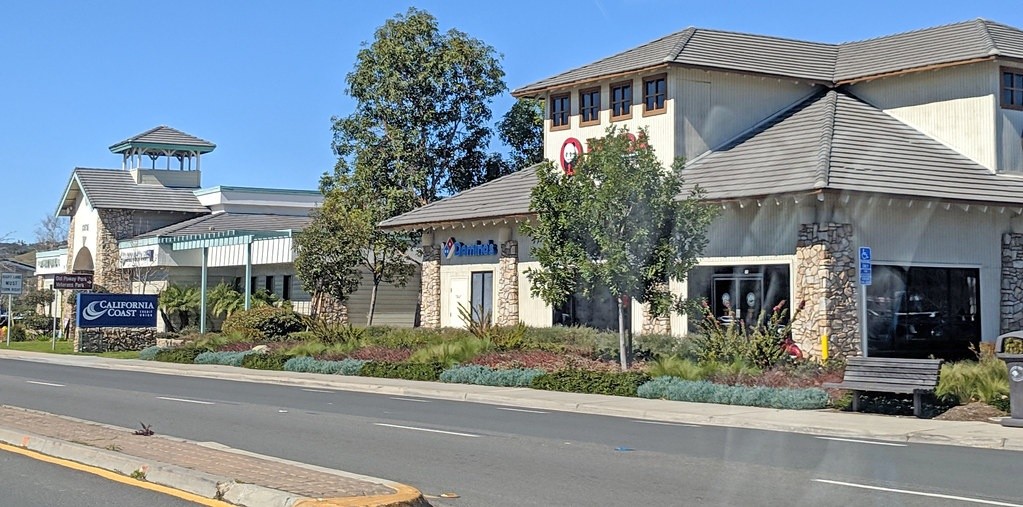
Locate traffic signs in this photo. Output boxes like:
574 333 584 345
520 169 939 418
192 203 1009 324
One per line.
53 273 93 290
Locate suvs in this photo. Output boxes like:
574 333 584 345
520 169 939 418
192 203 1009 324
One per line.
865 294 950 344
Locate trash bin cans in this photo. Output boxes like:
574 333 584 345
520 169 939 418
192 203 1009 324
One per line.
994 330 1023 428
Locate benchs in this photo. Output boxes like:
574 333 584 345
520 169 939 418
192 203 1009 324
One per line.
822 357 944 415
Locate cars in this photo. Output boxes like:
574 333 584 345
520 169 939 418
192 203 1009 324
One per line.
929 317 982 349
